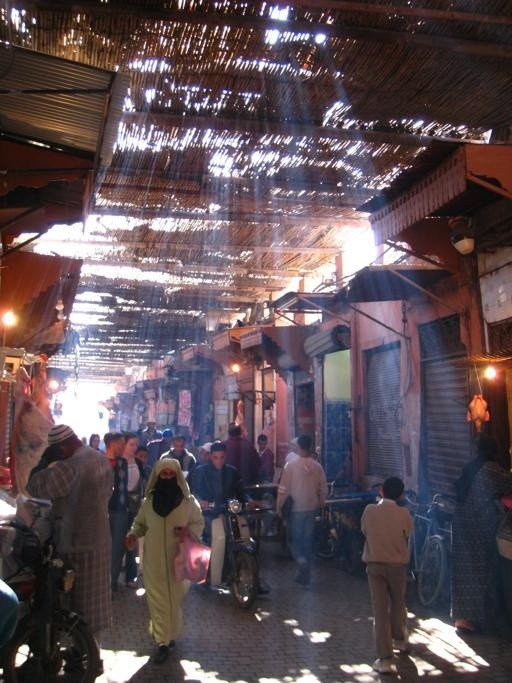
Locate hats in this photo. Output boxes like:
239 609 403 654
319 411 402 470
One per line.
197 442 213 454
48 425 75 445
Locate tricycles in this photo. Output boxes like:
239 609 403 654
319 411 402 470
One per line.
250 457 343 560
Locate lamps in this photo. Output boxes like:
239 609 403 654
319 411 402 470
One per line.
483 358 498 381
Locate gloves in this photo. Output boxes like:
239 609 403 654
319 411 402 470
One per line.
41 444 62 463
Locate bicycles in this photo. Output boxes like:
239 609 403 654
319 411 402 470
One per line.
390 487 447 606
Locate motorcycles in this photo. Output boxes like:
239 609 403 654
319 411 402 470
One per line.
1 514 100 682
183 495 273 611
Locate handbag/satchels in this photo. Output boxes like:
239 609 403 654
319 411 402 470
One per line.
282 496 293 518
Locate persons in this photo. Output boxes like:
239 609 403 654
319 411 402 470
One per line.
257 434 275 481
26 425 115 679
359 477 415 674
103 432 144 597
89 420 197 480
220 424 261 485
275 435 328 591
186 441 257 545
122 457 205 665
449 437 512 633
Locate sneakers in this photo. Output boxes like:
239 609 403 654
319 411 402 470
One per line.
256 578 271 593
295 563 310 585
454 618 475 632
373 658 391 674
393 628 409 652
155 640 174 664
